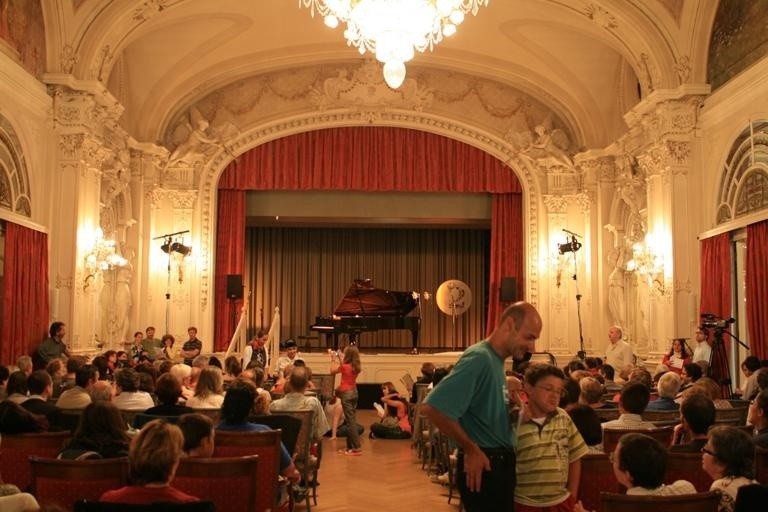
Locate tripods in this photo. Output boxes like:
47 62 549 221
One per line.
707 337 733 400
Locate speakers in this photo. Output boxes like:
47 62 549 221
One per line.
227 275 242 299
501 278 516 301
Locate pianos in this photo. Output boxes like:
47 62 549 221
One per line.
309 279 421 354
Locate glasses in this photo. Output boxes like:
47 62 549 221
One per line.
608 451 618 464
699 443 724 460
531 384 566 398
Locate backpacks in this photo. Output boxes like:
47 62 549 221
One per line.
380 416 402 429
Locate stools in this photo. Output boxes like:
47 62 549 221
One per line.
297 335 319 353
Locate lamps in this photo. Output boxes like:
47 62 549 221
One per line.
556 238 583 256
625 229 666 296
297 0 491 91
83 227 129 291
161 237 190 257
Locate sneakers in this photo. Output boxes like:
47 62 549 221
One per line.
337 444 363 457
436 471 452 484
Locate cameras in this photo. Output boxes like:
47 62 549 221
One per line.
336 352 339 356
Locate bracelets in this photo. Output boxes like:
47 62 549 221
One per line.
330 359 336 363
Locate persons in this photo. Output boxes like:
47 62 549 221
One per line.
411 362 453 403
507 358 765 511
369 391 412 441
324 384 365 441
421 301 542 511
0 321 330 512
376 381 399 423
328 344 364 456
692 325 714 365
605 325 634 377
660 340 691 373
